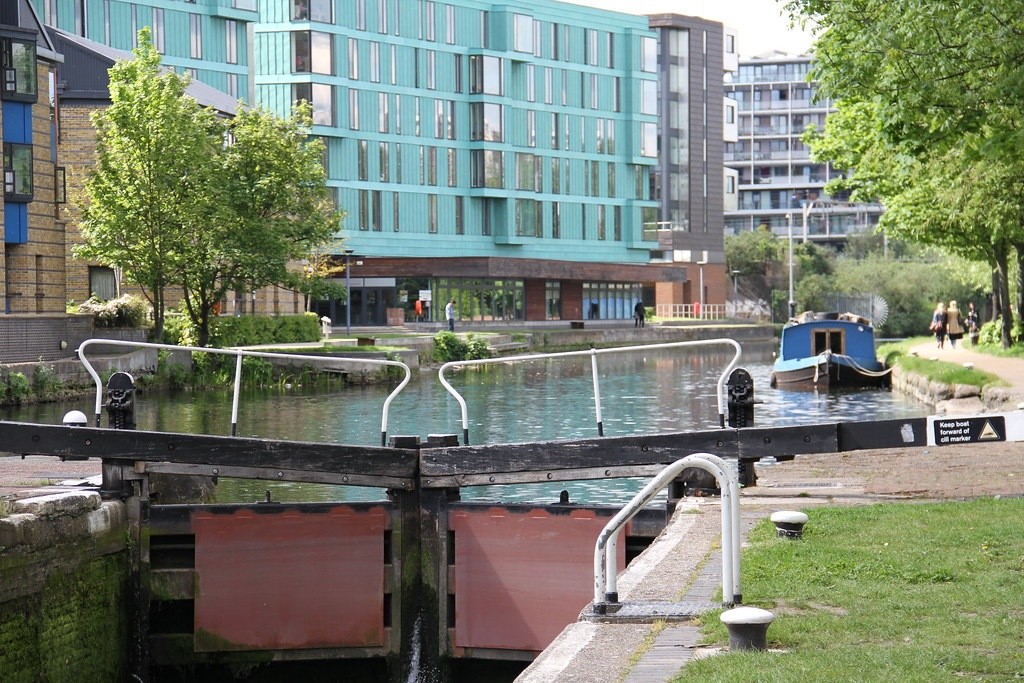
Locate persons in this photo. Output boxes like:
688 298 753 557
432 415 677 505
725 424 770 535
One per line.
635 299 646 328
445 300 456 332
931 299 979 348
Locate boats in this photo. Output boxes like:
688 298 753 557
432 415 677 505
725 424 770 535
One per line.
771 310 892 396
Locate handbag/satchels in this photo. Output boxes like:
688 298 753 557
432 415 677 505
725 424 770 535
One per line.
929 311 942 331
958 308 962 324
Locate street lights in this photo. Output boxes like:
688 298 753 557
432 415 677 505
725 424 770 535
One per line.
732 270 741 318
784 211 797 318
696 261 707 320
343 248 353 336
